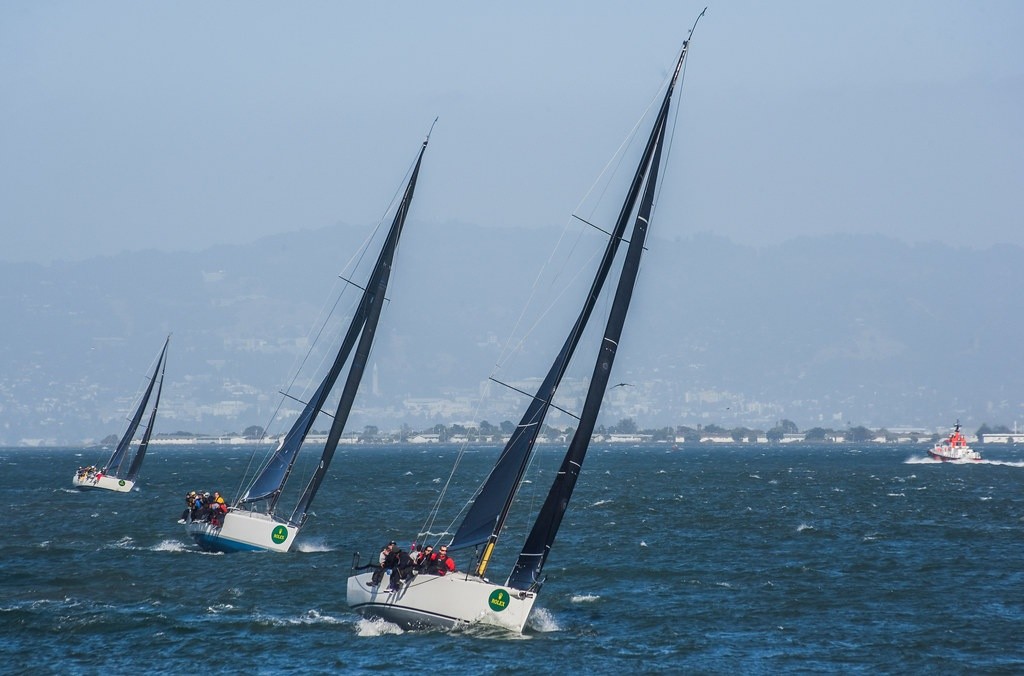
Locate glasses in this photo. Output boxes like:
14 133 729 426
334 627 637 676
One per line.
440 549 447 553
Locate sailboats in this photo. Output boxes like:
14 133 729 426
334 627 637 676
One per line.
348 6 713 638
176 114 440 547
72 333 171 493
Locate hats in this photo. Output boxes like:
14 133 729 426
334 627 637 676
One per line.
388 540 396 545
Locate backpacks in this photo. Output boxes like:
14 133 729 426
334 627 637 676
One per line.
428 554 448 576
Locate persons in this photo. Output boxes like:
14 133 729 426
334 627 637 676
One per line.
75 466 104 486
177 491 227 530
366 541 455 595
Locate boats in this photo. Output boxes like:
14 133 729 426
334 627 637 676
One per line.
926 418 982 461
